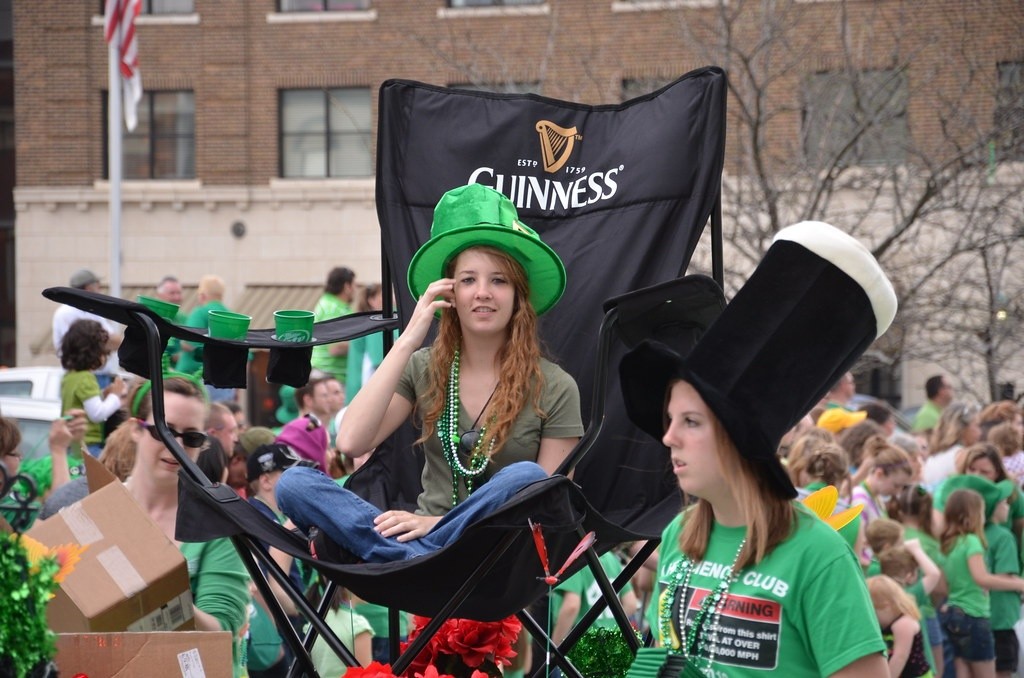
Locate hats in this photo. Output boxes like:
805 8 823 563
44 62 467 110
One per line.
70 269 99 287
619 221 897 502
816 408 867 433
933 475 1014 522
407 184 566 320
239 412 327 483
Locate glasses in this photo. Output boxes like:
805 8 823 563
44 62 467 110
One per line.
137 419 208 448
460 429 486 494
5 451 23 461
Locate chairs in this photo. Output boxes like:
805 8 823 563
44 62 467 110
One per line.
41 66 729 678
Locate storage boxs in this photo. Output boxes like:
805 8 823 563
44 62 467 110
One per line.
53 630 232 678
23 447 196 632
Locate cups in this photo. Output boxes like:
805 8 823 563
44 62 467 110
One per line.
273 310 315 343
137 295 181 324
207 310 252 342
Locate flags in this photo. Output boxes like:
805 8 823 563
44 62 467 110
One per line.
103 0 142 134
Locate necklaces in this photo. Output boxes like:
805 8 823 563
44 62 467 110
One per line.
436 343 496 508
661 537 747 673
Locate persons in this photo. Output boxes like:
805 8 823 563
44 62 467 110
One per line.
645 336 892 678
274 183 585 564
0 268 408 678
776 370 1024 678
503 539 658 678
